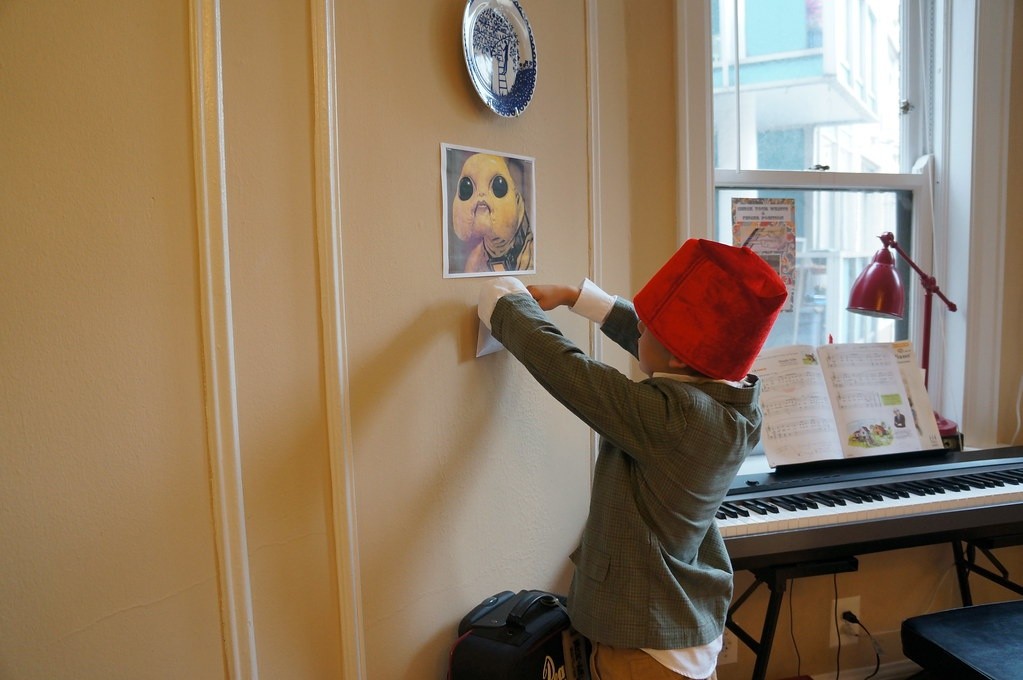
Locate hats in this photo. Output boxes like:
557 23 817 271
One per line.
632 239 788 381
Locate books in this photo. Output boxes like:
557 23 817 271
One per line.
752 339 945 469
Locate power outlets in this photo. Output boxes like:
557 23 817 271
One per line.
717 621 738 666
830 595 861 648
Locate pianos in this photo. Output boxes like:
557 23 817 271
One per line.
712 444 1023 574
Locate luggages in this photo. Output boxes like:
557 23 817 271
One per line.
447 589 592 680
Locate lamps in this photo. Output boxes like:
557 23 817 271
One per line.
845 229 966 452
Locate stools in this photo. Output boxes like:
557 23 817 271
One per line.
900 599 1023 680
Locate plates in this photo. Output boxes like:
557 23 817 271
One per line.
461 0 537 118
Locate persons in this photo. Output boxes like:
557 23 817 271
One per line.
479 240 787 679
